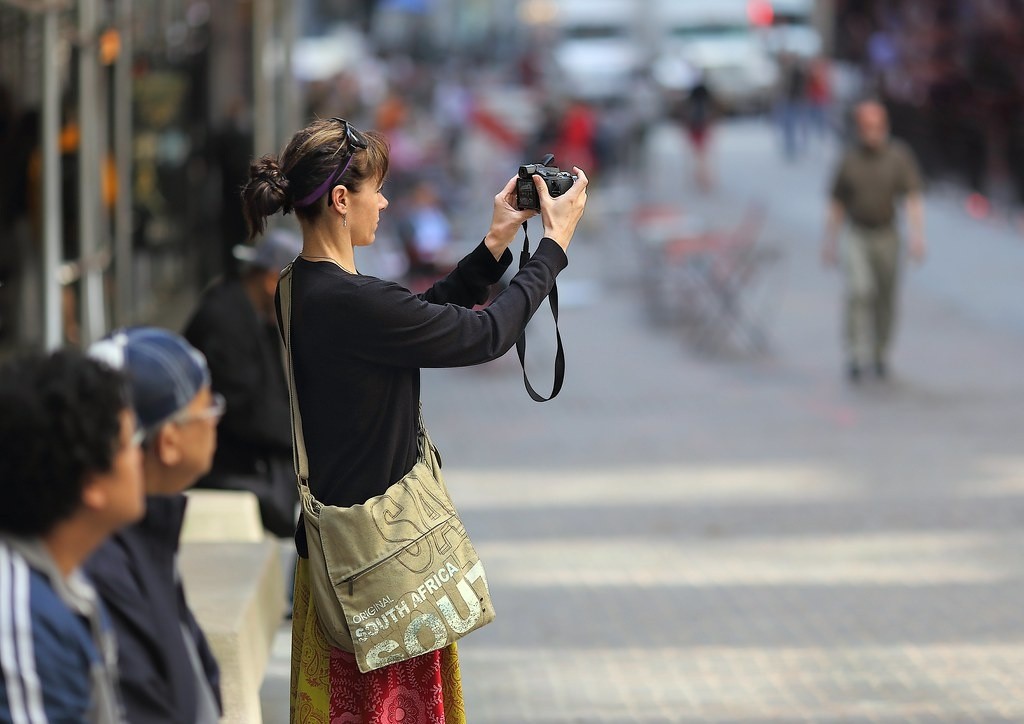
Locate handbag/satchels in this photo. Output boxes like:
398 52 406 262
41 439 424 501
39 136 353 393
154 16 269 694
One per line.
303 461 496 674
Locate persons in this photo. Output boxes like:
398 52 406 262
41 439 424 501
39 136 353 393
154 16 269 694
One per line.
822 100 925 384
182 227 301 538
243 118 588 704
1 350 146 724
78 327 224 723
296 39 807 364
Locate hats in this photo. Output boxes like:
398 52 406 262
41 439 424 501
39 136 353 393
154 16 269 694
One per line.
89 326 207 446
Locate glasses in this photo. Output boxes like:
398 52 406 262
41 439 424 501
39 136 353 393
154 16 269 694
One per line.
177 393 225 422
327 117 368 206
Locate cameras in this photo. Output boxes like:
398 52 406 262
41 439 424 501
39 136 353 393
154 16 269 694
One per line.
516 153 579 213
